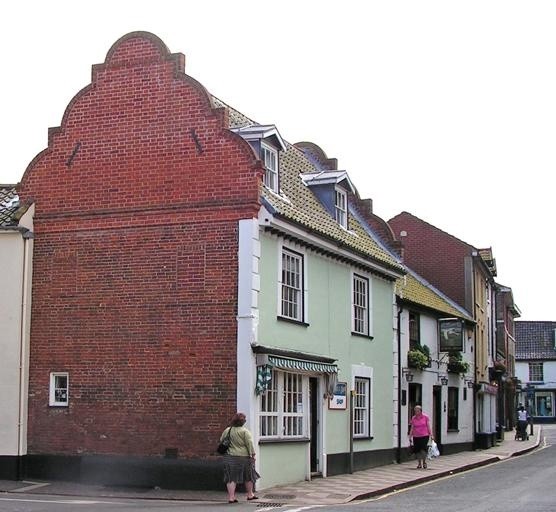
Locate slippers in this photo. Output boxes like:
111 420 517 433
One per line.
229 499 238 503
247 495 258 500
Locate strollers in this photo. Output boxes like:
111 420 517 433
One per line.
514 419 530 441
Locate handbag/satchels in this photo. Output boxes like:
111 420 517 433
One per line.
217 425 232 455
408 440 415 456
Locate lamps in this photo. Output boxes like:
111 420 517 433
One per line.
404 370 414 382
465 378 473 389
438 375 449 386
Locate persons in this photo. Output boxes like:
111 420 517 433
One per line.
219 412 261 503
409 405 432 469
515 401 529 442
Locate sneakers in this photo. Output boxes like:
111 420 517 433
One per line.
417 463 427 468
